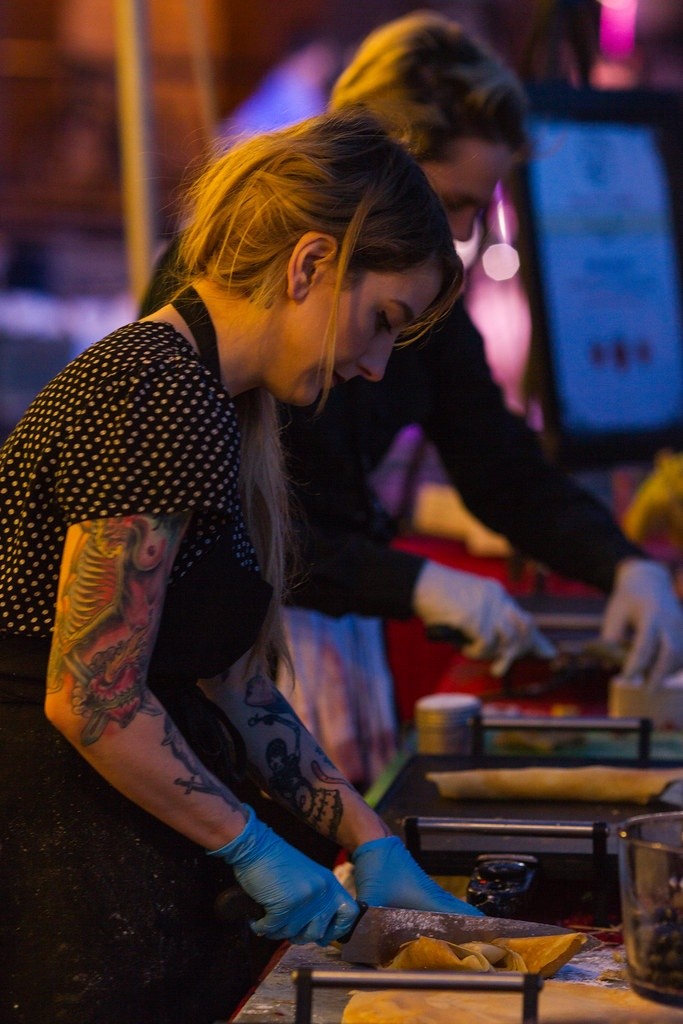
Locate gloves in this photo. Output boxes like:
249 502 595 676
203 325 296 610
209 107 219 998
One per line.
413 559 558 676
602 560 683 693
205 803 359 945
351 836 483 916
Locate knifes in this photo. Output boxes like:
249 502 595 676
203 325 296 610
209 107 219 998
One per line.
338 900 603 966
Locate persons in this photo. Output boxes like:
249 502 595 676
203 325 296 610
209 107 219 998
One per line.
0 116 487 1024
135 9 683 794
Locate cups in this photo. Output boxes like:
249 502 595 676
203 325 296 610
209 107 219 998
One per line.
415 692 485 757
614 809 683 1008
